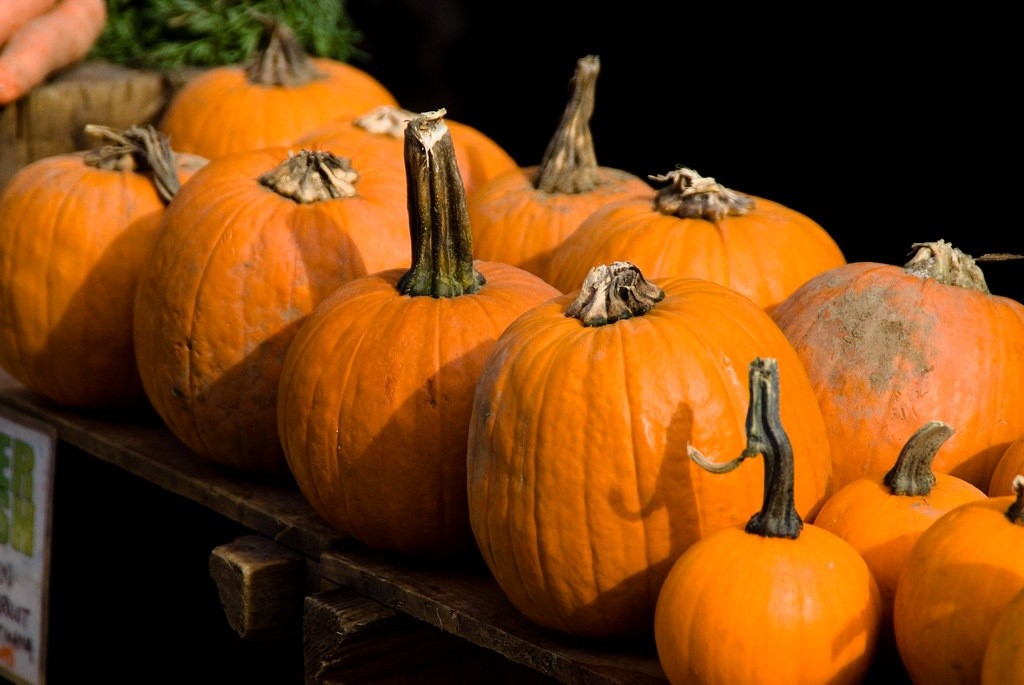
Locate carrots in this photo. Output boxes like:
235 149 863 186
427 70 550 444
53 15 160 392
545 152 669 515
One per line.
0 0 111 105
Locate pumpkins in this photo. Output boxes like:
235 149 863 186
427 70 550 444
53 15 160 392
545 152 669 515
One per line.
1 24 1023 685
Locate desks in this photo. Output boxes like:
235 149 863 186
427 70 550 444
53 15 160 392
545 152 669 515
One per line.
1 383 678 685
